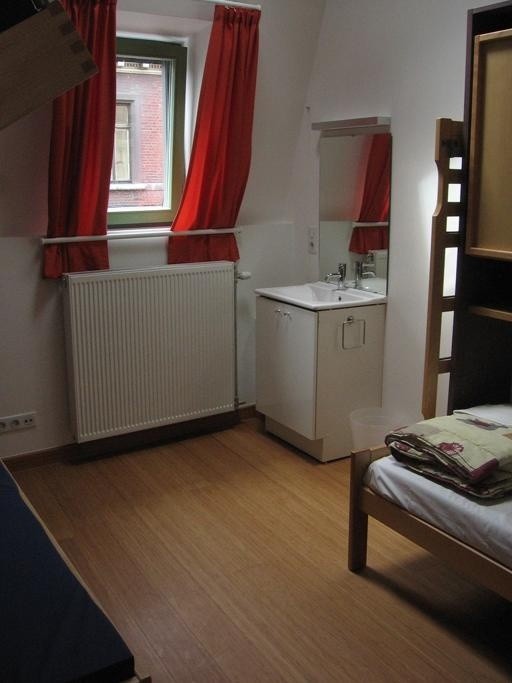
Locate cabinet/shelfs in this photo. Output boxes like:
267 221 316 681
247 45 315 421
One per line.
447 1 512 415
256 296 386 460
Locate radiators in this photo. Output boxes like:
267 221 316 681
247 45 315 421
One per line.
61 261 251 444
366 249 387 278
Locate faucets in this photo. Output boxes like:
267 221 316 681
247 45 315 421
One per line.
325 263 347 289
356 260 377 288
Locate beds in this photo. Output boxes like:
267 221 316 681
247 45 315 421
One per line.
348 445 512 603
1 453 153 683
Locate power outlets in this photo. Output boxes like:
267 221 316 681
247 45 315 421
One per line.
0 411 39 433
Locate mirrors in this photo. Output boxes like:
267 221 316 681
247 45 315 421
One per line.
318 133 391 296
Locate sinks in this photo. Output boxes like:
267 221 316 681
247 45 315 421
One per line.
347 277 386 296
253 283 372 308
